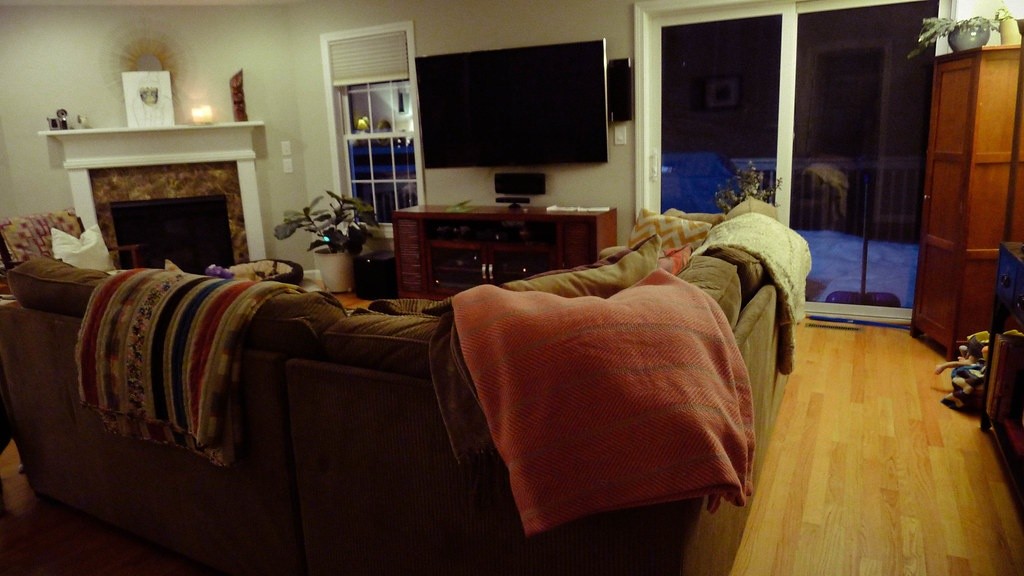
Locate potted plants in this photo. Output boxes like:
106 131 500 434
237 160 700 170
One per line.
271 191 381 293
906 9 1014 60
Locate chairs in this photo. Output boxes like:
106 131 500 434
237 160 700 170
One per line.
0 209 143 295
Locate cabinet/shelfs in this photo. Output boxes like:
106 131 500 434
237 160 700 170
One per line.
392 205 617 300
910 46 1024 362
980 241 1024 530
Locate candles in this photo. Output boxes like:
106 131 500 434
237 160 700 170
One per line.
192 104 212 123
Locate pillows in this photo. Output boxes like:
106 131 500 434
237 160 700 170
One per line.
630 206 712 251
661 208 727 226
49 223 117 273
500 233 661 299
656 244 694 276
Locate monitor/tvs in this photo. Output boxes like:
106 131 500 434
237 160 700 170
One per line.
414 37 608 169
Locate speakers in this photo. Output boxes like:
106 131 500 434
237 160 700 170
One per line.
607 57 632 122
495 173 545 195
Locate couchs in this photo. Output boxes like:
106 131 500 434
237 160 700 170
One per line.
0 199 811 576
